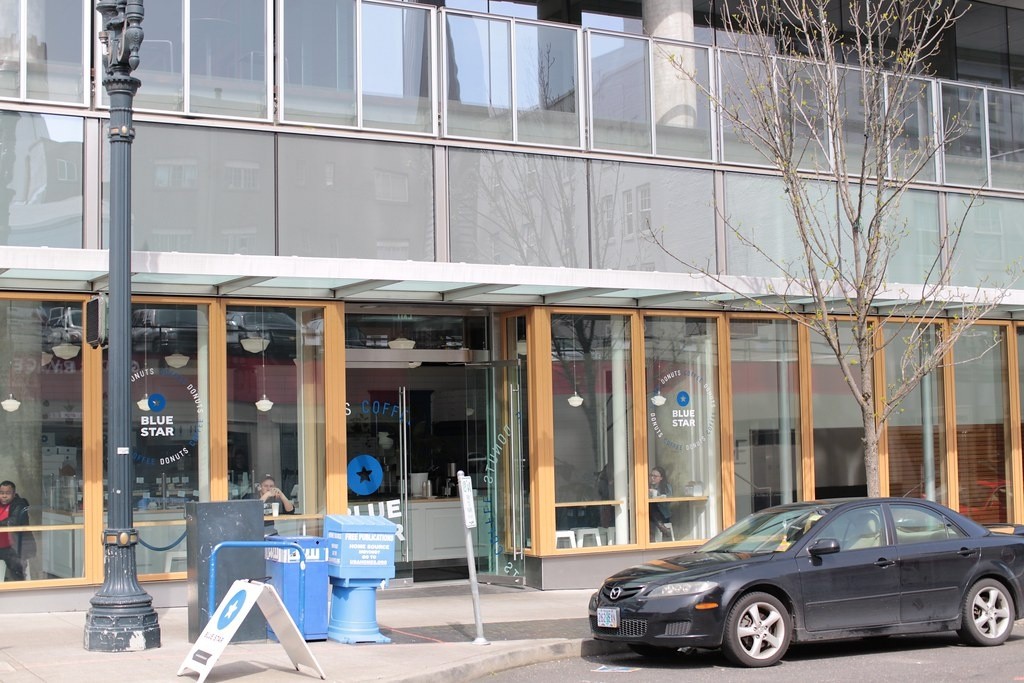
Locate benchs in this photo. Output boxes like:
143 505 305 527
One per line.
895 529 958 544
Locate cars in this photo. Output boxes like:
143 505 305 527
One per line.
587 495 1024 667
45 306 298 365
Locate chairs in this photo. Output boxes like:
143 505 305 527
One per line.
842 514 879 550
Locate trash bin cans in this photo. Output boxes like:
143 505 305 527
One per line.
265 536 328 643
185 500 267 644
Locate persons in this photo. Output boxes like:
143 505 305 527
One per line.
242 477 295 536
596 464 615 528
649 466 673 537
0 480 37 580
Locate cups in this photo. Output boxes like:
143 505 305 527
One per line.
272 503 279 517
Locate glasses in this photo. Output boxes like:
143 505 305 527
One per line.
650 474 661 478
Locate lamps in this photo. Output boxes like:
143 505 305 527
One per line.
164 306 190 368
136 305 155 411
567 318 585 407
651 358 666 406
1 301 21 412
51 304 79 360
240 306 270 353
388 314 415 348
255 307 274 411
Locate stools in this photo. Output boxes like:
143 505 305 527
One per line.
556 523 675 549
164 550 188 574
0 559 32 581
